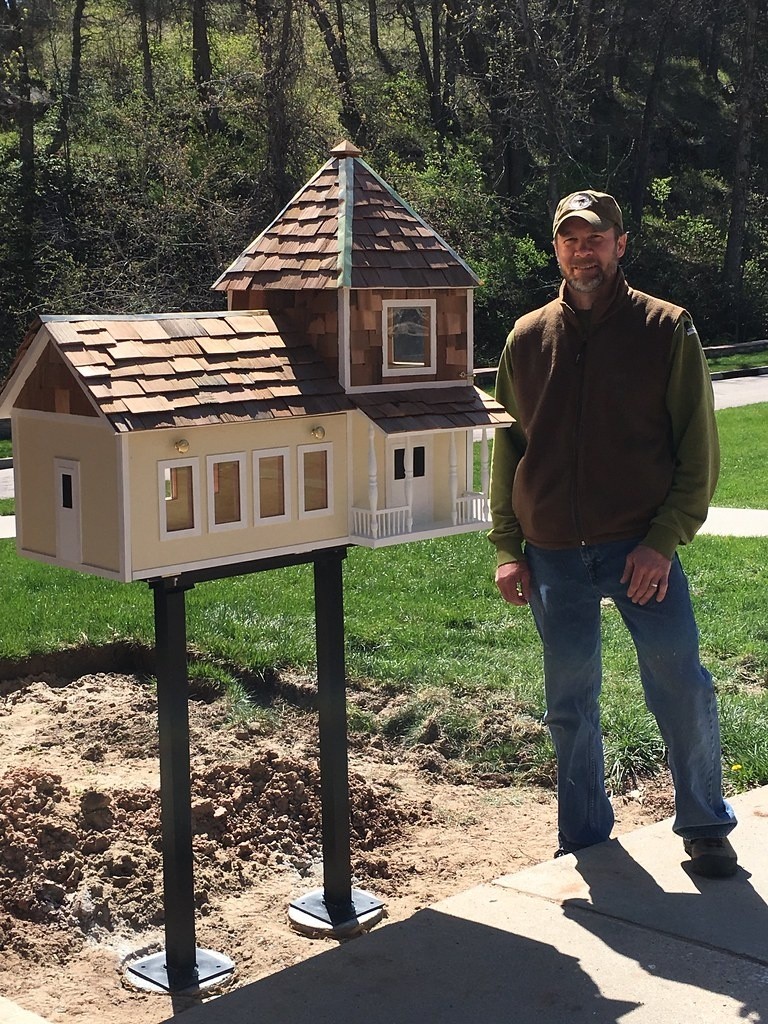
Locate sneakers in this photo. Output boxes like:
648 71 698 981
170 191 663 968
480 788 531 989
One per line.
683 836 738 876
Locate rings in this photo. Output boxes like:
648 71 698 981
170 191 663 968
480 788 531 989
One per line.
651 583 658 588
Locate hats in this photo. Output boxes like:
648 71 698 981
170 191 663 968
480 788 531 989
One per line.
552 190 624 238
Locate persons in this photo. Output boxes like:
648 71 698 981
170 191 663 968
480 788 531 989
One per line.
487 191 738 879
